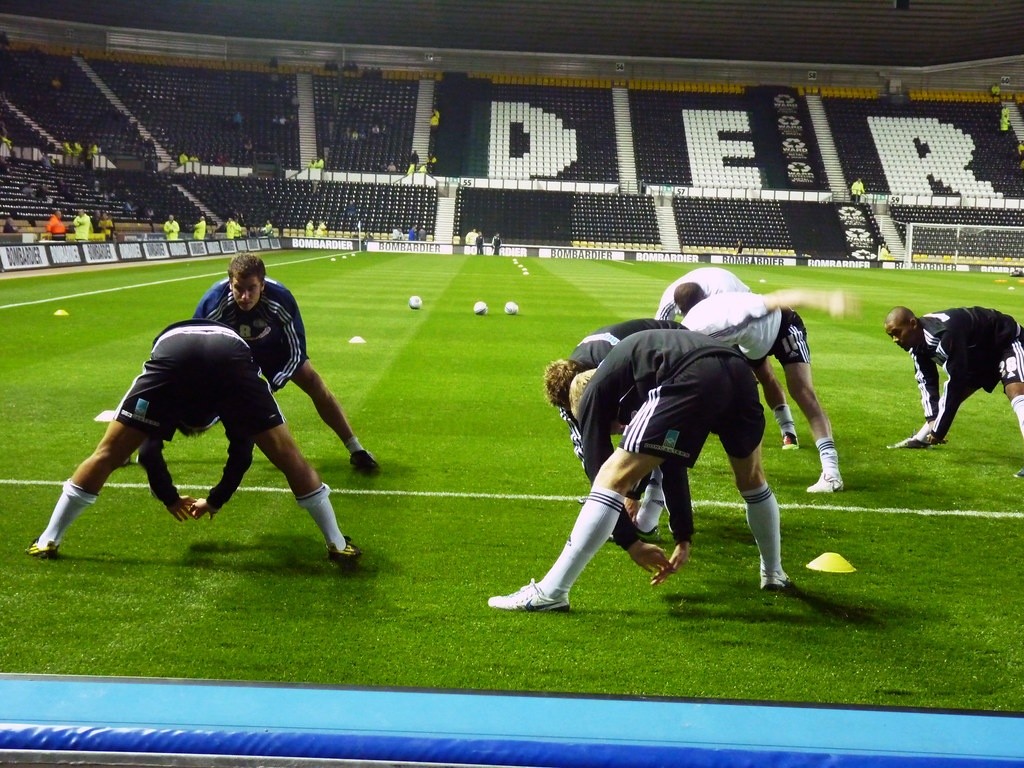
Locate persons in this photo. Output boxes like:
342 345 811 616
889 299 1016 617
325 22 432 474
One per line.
680 286 860 494
27 318 363 563
191 255 381 476
429 106 440 129
654 266 799 451
487 329 792 612
1000 104 1024 159
883 305 1024 477
992 82 1001 99
0 123 502 258
851 177 865 205
51 77 62 93
541 318 710 546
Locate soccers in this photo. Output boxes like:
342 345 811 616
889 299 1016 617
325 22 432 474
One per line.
474 301 488 315
505 301 519 315
409 295 423 309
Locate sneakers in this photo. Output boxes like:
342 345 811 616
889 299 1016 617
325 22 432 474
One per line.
760 570 790 589
781 431 799 450
887 437 928 451
606 522 659 543
24 537 59 560
488 577 570 613
806 474 844 494
325 535 361 561
350 451 380 473
